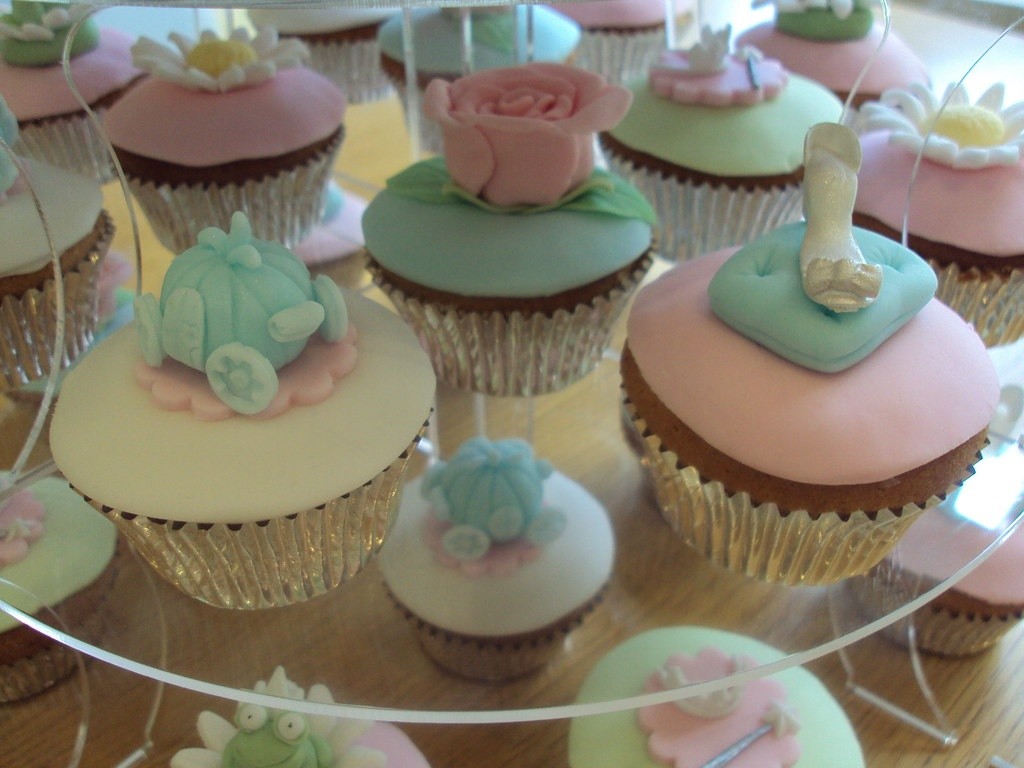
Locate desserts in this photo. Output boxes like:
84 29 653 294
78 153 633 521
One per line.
0 0 1024 768
